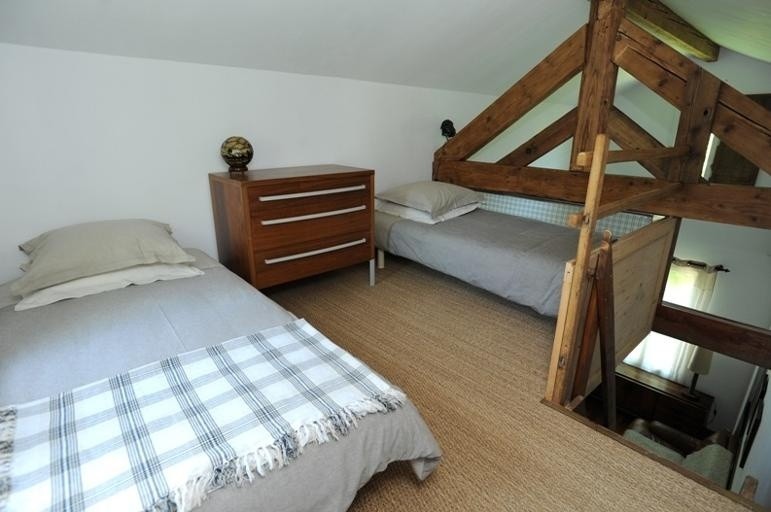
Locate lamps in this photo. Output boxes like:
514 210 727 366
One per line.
680 346 714 401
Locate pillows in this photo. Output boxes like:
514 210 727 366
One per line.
374 180 487 220
8 218 200 299
373 197 482 226
9 262 207 314
700 429 730 449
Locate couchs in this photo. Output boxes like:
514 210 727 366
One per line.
630 420 735 457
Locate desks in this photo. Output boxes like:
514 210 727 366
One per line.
207 163 377 291
585 361 717 442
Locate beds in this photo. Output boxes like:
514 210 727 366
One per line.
0 246 445 512
374 208 624 320
